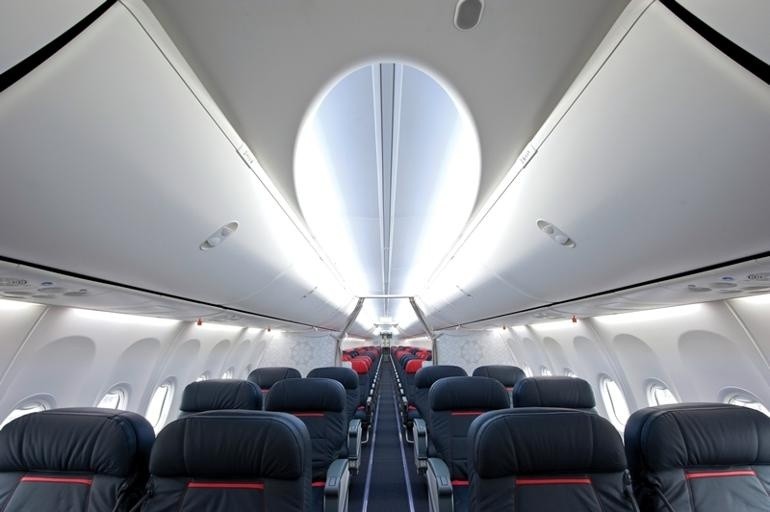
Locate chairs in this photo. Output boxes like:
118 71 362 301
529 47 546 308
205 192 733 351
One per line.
390 346 770 512
0 347 379 512
